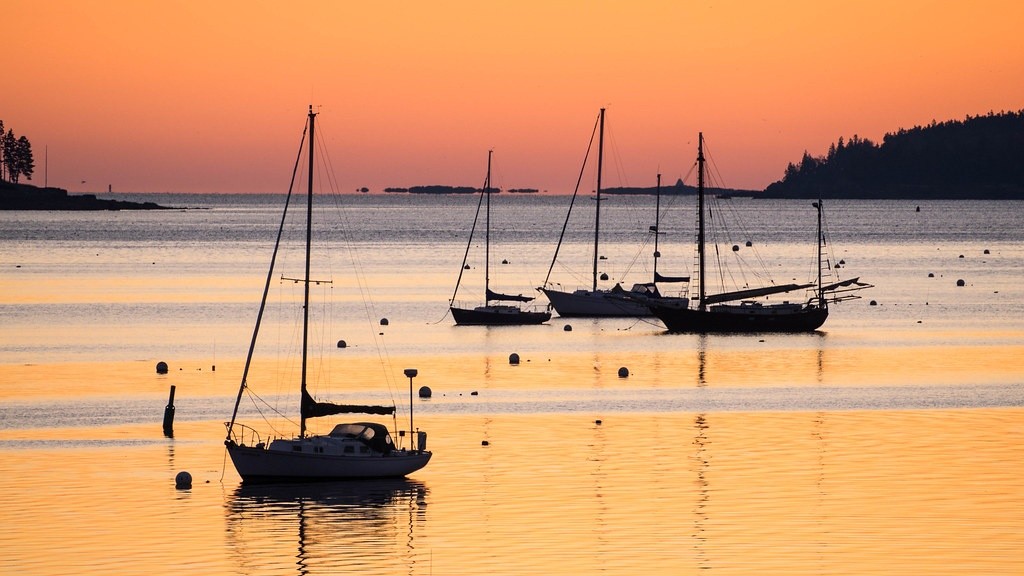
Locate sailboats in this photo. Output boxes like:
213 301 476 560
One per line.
537 109 689 317
649 132 875 332
450 151 552 324
224 104 433 480
